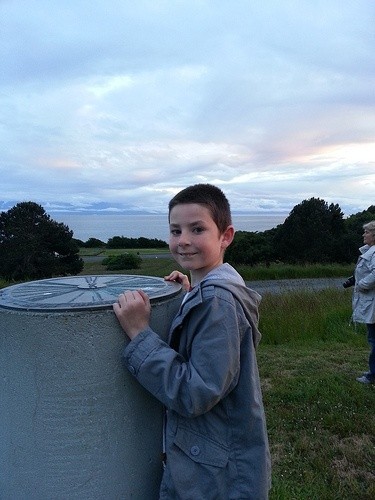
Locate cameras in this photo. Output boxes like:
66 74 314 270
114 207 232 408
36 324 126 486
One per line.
343 276 355 289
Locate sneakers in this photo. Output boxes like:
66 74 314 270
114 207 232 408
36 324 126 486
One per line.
356 371 372 383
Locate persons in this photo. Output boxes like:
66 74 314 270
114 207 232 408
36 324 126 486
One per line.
111 182 274 500
342 221 375 383
